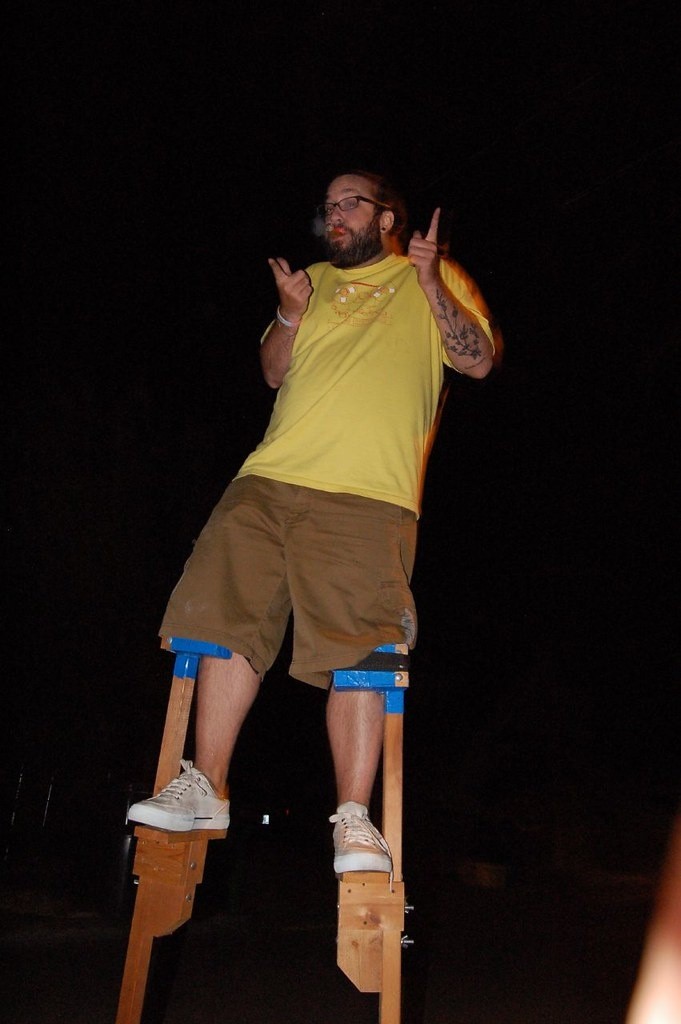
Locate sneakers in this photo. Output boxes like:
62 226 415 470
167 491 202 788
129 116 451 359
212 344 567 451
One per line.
329 801 393 892
126 759 230 831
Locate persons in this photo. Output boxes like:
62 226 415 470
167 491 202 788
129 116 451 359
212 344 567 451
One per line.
122 160 499 873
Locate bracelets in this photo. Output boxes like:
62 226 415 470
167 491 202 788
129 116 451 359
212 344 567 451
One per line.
276 305 302 328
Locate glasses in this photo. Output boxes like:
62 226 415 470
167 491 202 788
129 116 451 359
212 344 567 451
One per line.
316 196 394 218
